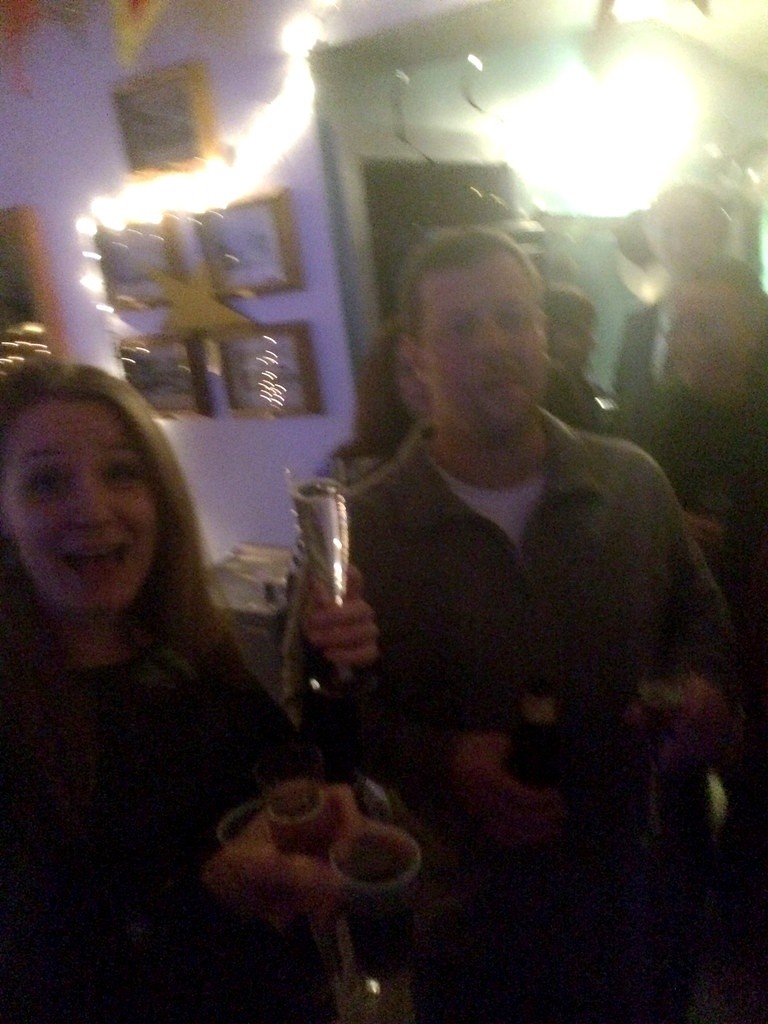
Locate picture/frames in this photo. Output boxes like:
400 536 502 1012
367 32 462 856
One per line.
89 205 190 312
119 330 213 421
212 319 325 417
192 189 308 299
110 57 222 180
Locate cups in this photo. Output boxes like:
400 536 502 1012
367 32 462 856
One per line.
294 473 353 608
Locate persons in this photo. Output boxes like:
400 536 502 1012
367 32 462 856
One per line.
0 356 367 1024
284 223 742 1022
308 183 767 601
